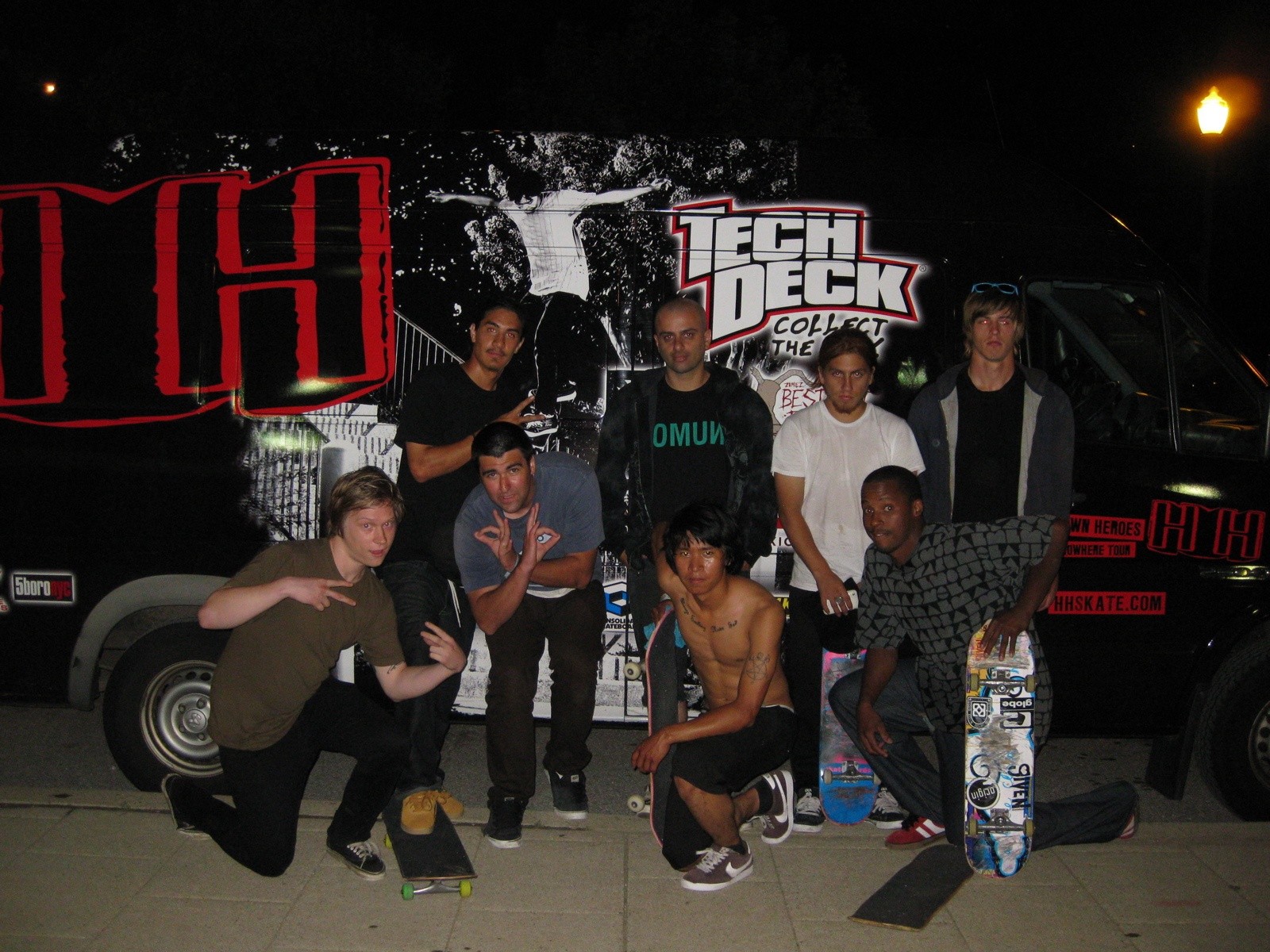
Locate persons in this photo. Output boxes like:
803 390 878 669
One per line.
594 298 779 817
454 421 606 852
826 464 1139 853
771 327 927 832
429 176 679 441
629 502 801 892
161 466 468 880
354 295 545 835
908 283 1076 611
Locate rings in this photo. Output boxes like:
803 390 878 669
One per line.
835 596 843 603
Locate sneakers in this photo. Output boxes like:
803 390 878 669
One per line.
400 792 437 835
544 764 590 820
430 788 467 818
745 770 795 844
884 814 945 850
681 841 754 892
863 785 904 830
792 786 826 833
1121 812 1137 838
325 835 386 880
161 773 211 840
484 794 529 851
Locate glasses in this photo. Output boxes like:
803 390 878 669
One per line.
970 282 1020 297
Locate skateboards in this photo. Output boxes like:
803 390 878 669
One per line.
809 599 881 826
964 619 1036 878
380 790 478 901
625 610 680 847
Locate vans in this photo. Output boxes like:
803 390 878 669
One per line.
1 137 1268 820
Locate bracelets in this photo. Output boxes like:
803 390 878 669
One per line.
508 553 522 574
471 431 476 439
